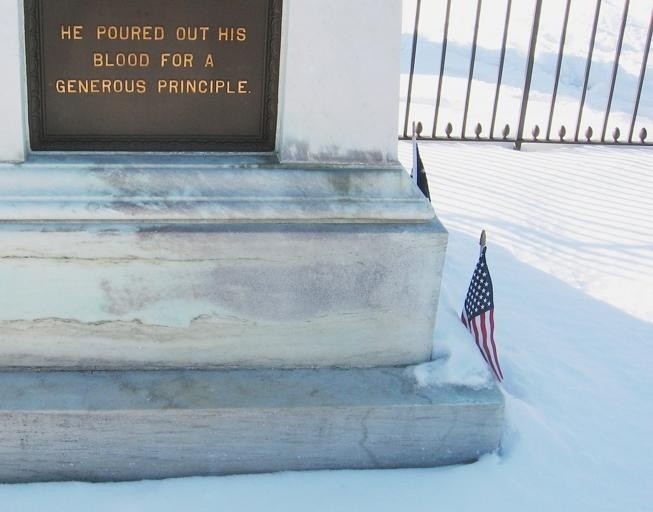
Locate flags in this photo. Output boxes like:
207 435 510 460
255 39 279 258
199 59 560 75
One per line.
410 141 431 203
460 245 503 382
